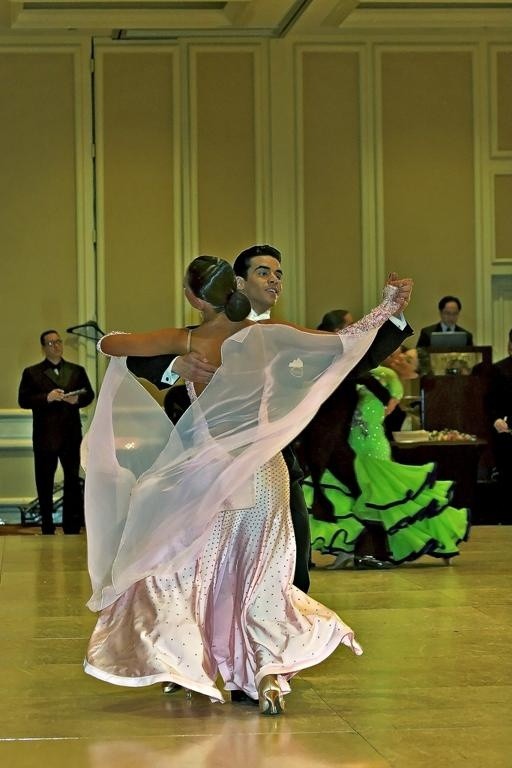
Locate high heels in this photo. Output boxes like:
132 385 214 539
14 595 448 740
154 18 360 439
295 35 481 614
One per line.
256 673 287 717
323 551 354 569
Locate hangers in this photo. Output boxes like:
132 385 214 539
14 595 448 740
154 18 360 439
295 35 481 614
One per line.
65 312 107 342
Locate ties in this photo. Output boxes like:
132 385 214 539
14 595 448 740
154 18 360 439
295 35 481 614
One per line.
446 327 451 332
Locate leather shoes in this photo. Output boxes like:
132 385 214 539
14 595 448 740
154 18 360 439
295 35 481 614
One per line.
231 689 248 702
353 554 395 570
161 680 183 693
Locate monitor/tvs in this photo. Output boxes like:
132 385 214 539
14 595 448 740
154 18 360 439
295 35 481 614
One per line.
430 332 468 347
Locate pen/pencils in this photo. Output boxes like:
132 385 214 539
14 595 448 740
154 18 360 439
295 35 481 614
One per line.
504 416 507 423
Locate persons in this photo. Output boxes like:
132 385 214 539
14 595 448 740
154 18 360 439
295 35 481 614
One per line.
122 246 410 705
18 329 95 535
80 254 413 719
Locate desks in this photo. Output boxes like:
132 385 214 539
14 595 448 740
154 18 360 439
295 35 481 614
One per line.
389 429 487 542
